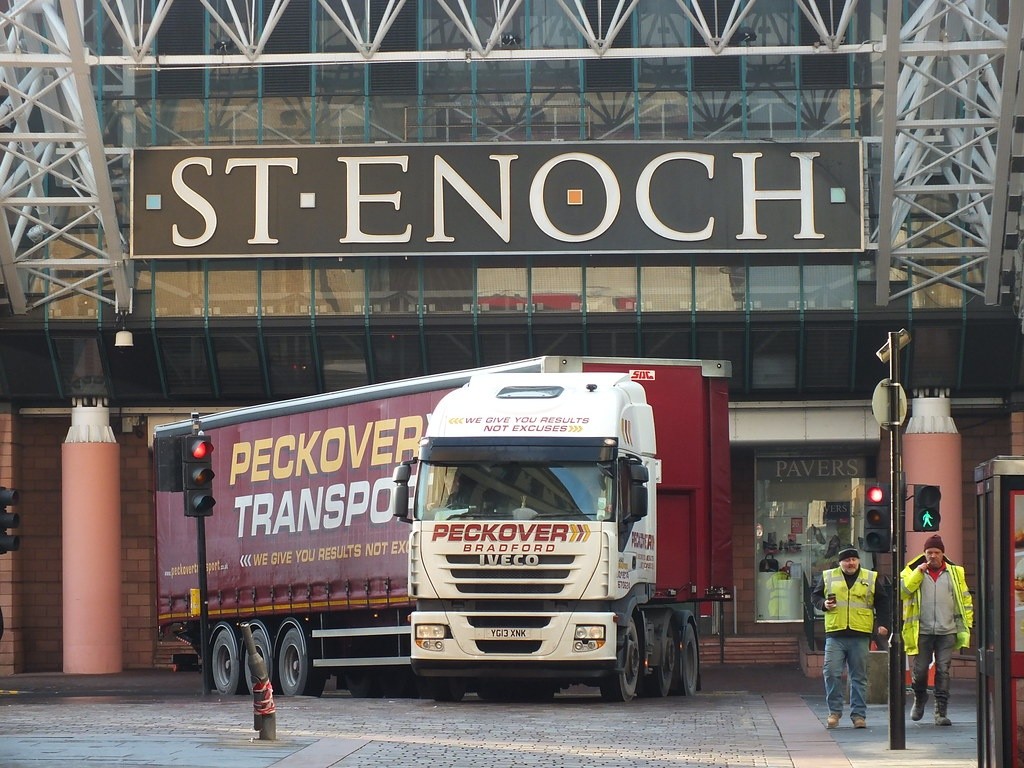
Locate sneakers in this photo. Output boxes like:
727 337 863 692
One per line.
853 718 865 728
827 713 840 726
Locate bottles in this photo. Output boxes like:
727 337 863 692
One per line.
827 593 837 608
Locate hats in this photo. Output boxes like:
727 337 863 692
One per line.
837 544 859 562
923 534 944 553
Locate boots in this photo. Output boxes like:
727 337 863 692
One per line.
933 703 951 725
910 690 929 721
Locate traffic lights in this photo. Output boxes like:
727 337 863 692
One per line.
181 434 216 517
913 484 941 532
863 483 891 553
0 487 20 554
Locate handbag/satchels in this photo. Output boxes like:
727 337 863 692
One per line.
780 560 794 573
759 554 778 572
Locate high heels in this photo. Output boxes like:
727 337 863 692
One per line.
824 535 841 559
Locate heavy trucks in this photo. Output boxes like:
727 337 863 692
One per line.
151 352 734 703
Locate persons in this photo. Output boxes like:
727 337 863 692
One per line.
766 569 796 619
900 534 973 725
811 543 889 729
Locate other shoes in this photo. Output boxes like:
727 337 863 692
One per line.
814 528 825 544
779 539 803 551
